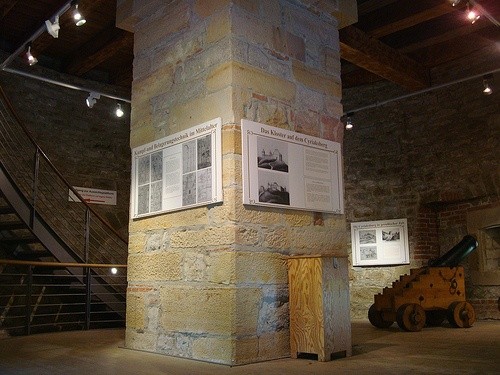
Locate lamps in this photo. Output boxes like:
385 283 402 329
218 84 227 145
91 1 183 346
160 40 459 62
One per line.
346 112 354 129
482 74 492 96
45 16 60 37
86 92 100 109
71 0 87 26
25 40 38 66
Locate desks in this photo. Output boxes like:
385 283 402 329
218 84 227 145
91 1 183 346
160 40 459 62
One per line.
283 254 352 361
